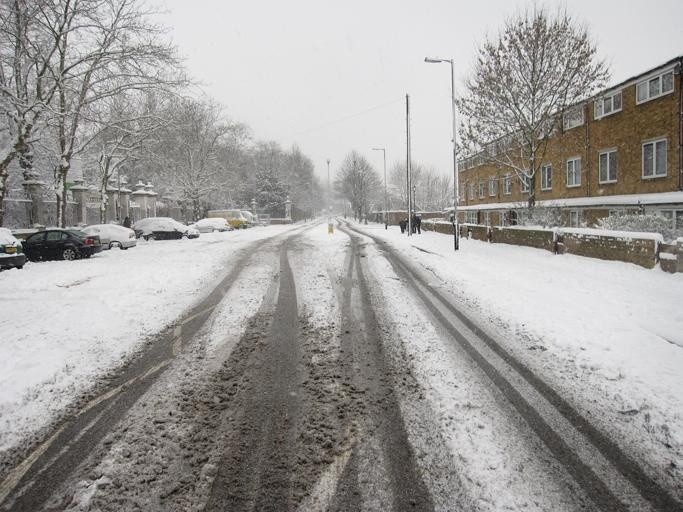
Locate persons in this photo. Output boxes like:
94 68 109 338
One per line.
399 214 421 234
123 216 131 228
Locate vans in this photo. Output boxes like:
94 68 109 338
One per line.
207 209 257 230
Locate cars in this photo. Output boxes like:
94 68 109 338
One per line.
0 216 231 272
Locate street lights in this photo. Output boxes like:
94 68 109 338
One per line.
372 148 387 230
327 158 331 184
425 57 459 249
